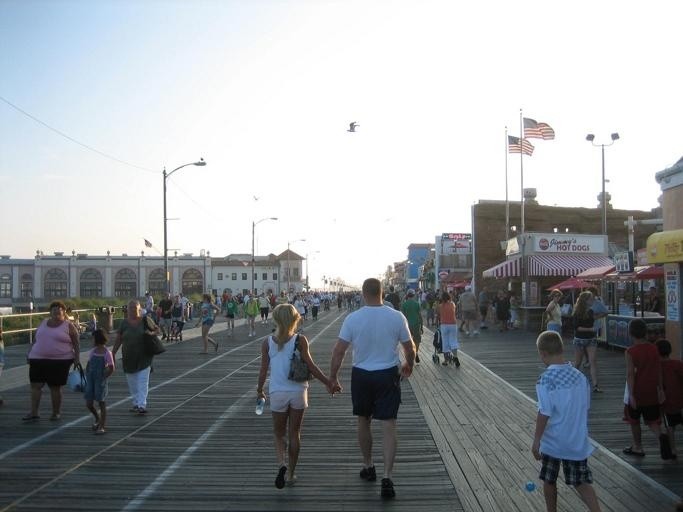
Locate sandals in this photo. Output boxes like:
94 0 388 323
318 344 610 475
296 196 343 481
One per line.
453 357 461 368
273 465 287 490
442 361 449 366
90 418 106 435
592 384 604 394
285 475 298 487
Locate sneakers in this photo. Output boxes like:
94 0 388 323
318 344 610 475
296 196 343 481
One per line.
248 330 257 337
457 326 490 337
415 354 420 364
359 466 377 483
380 478 397 501
261 319 268 325
161 333 183 343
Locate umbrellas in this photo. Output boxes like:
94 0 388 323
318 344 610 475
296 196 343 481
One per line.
544 276 595 306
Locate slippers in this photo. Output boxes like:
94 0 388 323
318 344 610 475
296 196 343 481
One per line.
623 446 647 456
658 433 672 461
199 342 219 355
22 415 41 420
130 404 147 414
49 413 61 420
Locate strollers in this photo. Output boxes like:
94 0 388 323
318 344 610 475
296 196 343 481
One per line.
430 327 455 367
166 321 187 344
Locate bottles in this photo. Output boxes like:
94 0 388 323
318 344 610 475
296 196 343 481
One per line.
256 397 267 415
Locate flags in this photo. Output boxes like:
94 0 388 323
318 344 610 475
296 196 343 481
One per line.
507 135 534 156
144 239 153 249
523 117 555 140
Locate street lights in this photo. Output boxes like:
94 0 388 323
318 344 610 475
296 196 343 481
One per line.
246 214 280 297
320 274 359 292
305 250 322 292
162 156 207 295
585 130 620 232
285 239 307 288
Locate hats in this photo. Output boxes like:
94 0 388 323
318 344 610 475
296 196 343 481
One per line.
406 288 416 296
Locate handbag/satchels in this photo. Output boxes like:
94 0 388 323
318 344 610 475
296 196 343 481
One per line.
143 315 168 357
67 360 88 393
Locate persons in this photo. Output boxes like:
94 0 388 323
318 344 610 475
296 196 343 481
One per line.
65 306 74 320
326 277 418 500
532 329 601 512
569 291 604 394
435 292 461 369
88 314 97 331
83 327 115 434
255 303 344 491
22 300 81 421
381 285 522 364
112 298 168 415
144 292 155 312
192 293 221 356
646 286 660 312
620 316 673 461
151 289 363 344
586 286 611 340
655 338 683 458
545 289 564 336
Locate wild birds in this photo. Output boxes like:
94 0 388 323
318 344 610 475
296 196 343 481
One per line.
346 121 360 133
